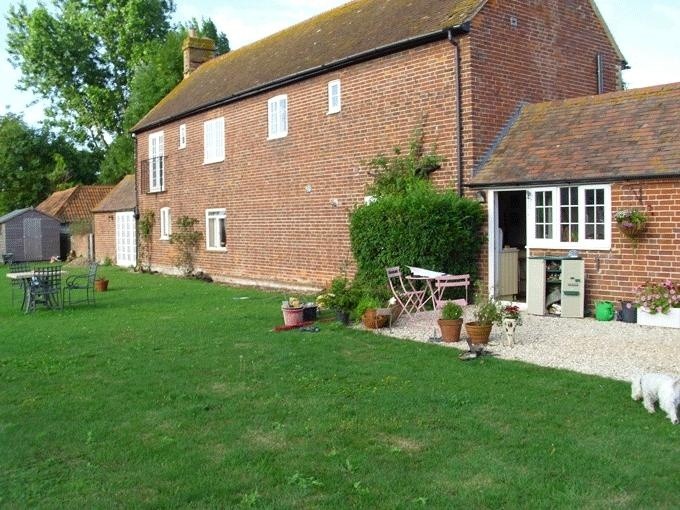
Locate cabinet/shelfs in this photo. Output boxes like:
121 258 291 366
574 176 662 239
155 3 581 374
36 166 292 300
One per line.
498 247 520 301
526 254 585 317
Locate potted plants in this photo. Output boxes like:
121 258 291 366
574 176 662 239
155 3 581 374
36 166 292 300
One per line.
316 274 360 326
435 299 465 343
464 274 504 346
355 291 387 329
95 274 109 293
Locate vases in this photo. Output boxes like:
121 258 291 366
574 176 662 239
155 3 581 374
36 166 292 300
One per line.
619 224 644 237
502 319 519 346
636 306 680 328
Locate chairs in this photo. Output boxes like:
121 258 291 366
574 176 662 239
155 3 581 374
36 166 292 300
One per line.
386 264 427 325
434 270 471 312
4 260 100 316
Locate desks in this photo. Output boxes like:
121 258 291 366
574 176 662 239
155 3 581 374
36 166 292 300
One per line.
405 273 451 321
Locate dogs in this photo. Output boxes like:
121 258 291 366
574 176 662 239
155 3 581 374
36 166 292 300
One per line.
630 371 680 424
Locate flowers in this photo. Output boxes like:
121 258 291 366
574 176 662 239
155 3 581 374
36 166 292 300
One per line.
501 302 523 326
622 277 680 315
613 206 648 232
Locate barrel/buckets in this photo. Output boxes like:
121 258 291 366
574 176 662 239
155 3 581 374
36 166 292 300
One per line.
619 300 637 322
281 307 304 325
592 300 614 321
94 280 109 294
304 307 318 322
